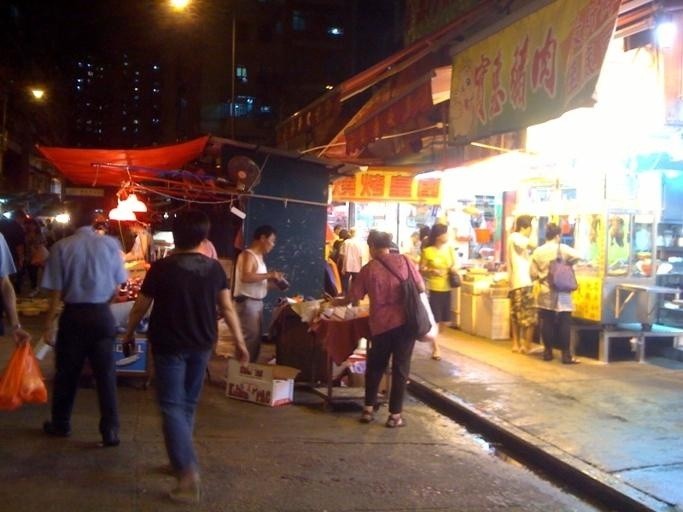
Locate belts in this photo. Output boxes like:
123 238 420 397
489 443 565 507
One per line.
232 294 265 303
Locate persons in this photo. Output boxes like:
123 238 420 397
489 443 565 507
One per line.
122 208 251 501
233 222 284 364
0 229 32 346
330 210 473 428
506 214 532 352
606 216 629 265
0 208 70 297
40 203 123 449
527 220 584 364
198 239 219 260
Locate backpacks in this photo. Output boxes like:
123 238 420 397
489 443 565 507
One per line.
373 253 433 340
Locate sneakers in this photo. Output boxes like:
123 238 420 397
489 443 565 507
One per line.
166 482 202 507
40 416 73 438
100 428 121 448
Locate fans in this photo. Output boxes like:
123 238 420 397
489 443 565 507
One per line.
224 153 261 198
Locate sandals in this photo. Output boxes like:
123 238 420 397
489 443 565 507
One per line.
357 408 376 425
561 356 581 364
384 414 408 428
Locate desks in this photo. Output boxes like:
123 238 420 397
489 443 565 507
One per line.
275 296 393 413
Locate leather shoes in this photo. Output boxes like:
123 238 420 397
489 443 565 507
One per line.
431 351 442 362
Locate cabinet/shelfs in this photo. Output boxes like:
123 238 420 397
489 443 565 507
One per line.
632 218 682 325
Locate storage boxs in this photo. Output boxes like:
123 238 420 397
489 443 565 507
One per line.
344 360 391 395
447 286 510 341
224 358 301 408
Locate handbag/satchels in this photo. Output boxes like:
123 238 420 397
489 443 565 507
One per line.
447 268 462 288
546 242 578 294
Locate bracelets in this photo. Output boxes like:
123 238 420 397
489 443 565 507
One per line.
13 324 21 329
